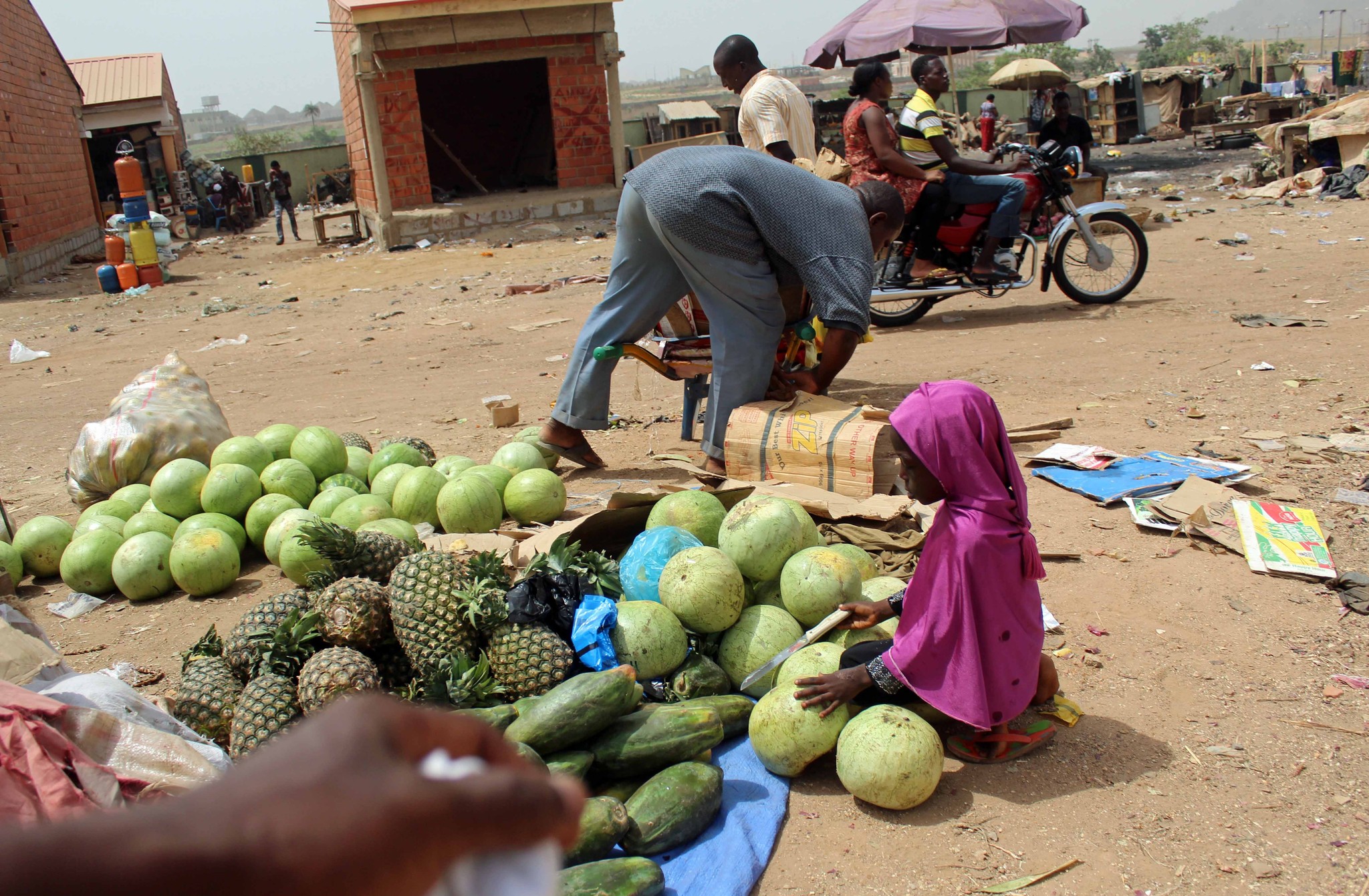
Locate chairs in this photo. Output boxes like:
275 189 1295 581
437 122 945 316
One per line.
208 197 227 230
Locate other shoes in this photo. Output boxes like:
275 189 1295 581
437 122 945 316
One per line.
295 237 301 240
276 240 284 244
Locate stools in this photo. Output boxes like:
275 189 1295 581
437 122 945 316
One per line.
103 211 116 228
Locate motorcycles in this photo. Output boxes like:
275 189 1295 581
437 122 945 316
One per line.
843 143 1158 329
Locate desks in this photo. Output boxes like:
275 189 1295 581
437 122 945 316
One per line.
1061 176 1103 207
1281 127 1312 177
1191 119 1267 148
1248 97 1302 121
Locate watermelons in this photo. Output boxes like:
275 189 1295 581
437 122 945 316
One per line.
0 421 566 601
607 489 944 810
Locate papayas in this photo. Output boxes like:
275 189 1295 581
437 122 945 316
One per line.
436 664 757 896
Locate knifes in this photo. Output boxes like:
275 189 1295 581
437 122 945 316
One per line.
740 608 853 692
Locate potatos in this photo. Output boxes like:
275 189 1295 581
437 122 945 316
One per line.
69 358 233 508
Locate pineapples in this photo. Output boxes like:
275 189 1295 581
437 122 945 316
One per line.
173 518 578 763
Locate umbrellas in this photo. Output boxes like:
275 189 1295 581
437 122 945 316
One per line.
802 0 1091 158
987 58 1071 117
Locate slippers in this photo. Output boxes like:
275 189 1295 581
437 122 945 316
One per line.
947 720 1057 763
970 265 1022 280
912 267 965 281
537 441 601 469
705 477 721 487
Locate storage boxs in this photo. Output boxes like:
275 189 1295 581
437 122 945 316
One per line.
142 175 160 214
129 126 151 144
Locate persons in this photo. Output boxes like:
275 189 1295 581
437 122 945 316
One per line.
793 380 1059 763
883 104 896 132
978 91 1000 154
1025 88 1110 211
266 159 301 245
0 672 590 896
535 144 906 476
841 57 957 280
895 54 1032 281
712 34 817 175
209 167 258 235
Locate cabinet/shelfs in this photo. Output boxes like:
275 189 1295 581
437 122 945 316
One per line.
242 180 268 217
1084 76 1139 144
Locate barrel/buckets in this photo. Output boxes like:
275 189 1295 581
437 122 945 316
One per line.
170 215 190 239
242 165 254 183
